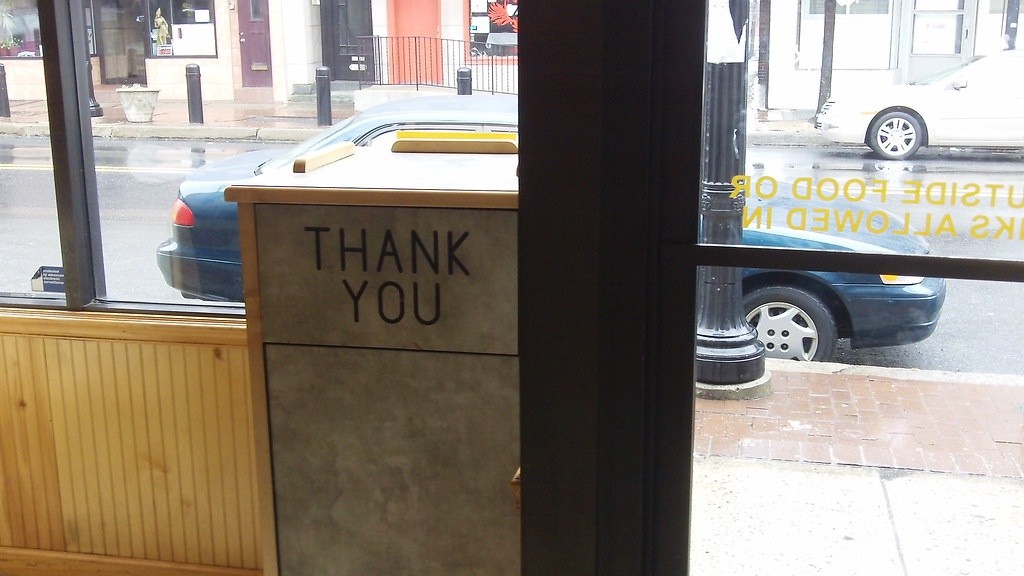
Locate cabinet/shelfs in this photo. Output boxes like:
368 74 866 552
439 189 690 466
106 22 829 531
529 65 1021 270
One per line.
223 142 522 576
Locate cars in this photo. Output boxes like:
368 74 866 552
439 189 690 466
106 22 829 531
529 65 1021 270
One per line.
814 48 1022 162
156 93 948 364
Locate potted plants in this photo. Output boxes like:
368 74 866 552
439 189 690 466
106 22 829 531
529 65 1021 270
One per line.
116 83 161 122
